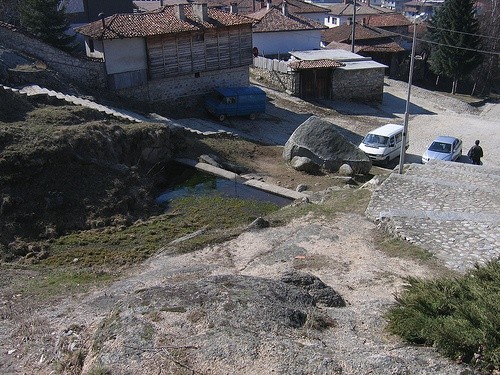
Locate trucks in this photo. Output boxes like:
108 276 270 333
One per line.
205 87 266 121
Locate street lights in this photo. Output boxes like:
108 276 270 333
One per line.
398 13 430 173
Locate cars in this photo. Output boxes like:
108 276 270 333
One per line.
422 136 462 164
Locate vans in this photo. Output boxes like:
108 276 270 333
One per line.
358 124 410 167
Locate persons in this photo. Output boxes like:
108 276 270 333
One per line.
468 140 484 166
